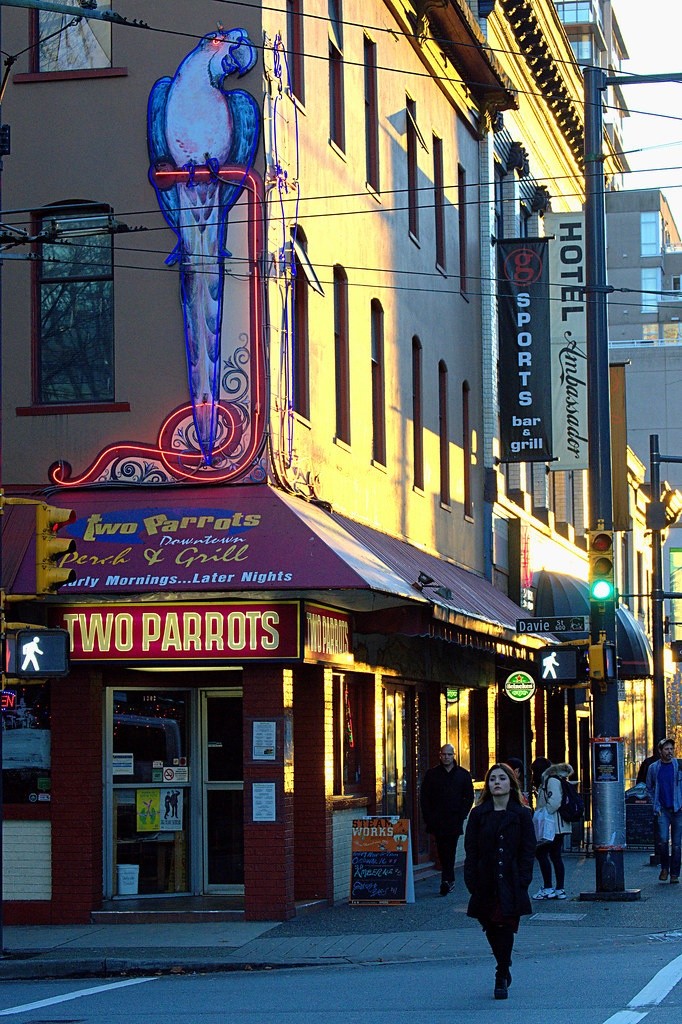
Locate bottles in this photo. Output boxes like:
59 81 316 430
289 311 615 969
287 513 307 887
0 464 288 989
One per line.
355 763 361 783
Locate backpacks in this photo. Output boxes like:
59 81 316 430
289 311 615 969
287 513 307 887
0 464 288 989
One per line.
544 775 585 823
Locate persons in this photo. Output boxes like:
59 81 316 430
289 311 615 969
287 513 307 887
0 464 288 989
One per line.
505 758 586 900
647 738 682 885
465 763 537 1000
419 745 475 897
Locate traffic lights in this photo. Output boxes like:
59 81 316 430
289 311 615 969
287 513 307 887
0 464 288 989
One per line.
537 644 581 683
588 530 620 603
16 628 70 676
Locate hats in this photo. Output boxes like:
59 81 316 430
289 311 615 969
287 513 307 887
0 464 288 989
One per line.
658 739 675 748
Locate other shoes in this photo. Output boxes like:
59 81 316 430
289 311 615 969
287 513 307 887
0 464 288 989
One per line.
439 878 455 897
659 868 669 881
670 874 679 884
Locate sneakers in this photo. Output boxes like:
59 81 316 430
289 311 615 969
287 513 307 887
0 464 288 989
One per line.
532 885 554 900
543 887 567 901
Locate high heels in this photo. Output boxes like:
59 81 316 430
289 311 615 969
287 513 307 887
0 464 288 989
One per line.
495 960 513 1000
